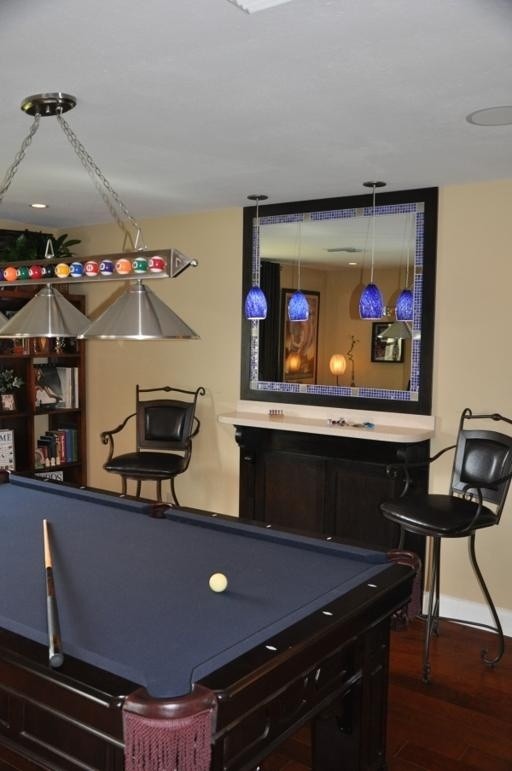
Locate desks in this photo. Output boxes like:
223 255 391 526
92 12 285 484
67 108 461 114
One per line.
0 469 421 771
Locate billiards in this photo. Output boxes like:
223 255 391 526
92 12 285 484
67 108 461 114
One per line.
0 255 167 282
209 572 227 593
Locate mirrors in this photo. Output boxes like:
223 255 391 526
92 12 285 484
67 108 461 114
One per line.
371 322 405 363
239 186 439 416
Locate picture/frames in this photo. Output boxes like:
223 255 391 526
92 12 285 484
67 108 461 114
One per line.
279 288 320 384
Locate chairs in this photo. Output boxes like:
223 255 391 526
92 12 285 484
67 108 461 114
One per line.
379 408 512 685
99 384 205 506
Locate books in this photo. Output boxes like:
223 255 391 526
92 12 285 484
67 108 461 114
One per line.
0 429 16 472
33 367 81 468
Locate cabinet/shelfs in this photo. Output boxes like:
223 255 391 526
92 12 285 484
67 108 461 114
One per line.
234 424 430 632
0 296 88 493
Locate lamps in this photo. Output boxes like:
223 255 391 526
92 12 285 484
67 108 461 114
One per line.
330 354 346 386
395 212 413 322
0 93 201 341
245 179 386 321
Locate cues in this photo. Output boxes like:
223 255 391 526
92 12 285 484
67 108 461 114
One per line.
42 518 64 667
